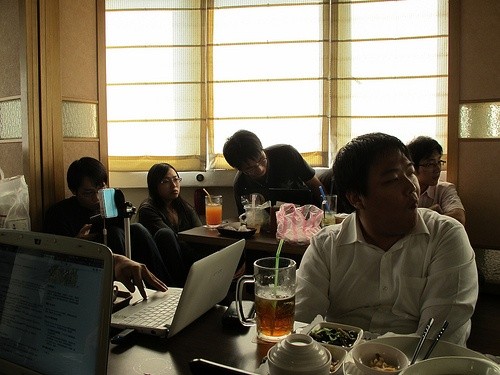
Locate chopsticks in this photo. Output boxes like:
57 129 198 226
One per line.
409 318 449 366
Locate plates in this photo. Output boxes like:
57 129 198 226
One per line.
342 337 496 375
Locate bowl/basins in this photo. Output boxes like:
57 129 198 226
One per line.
267 334 332 375
398 356 500 375
351 342 409 375
306 321 364 374
334 214 349 224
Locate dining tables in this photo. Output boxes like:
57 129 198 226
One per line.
178 216 308 255
108 280 500 375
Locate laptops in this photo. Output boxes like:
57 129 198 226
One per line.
0 227 111 375
110 239 245 337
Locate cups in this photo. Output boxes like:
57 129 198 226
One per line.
244 204 264 233
320 195 337 228
236 257 297 342
205 195 223 225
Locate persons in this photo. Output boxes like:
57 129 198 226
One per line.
317 168 356 214
138 163 203 288
406 136 465 226
44 157 174 287
113 253 168 299
223 130 328 221
294 134 478 347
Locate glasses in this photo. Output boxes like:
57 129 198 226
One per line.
415 160 446 169
237 151 266 173
160 178 181 184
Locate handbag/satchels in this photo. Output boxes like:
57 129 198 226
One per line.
275 202 324 246
